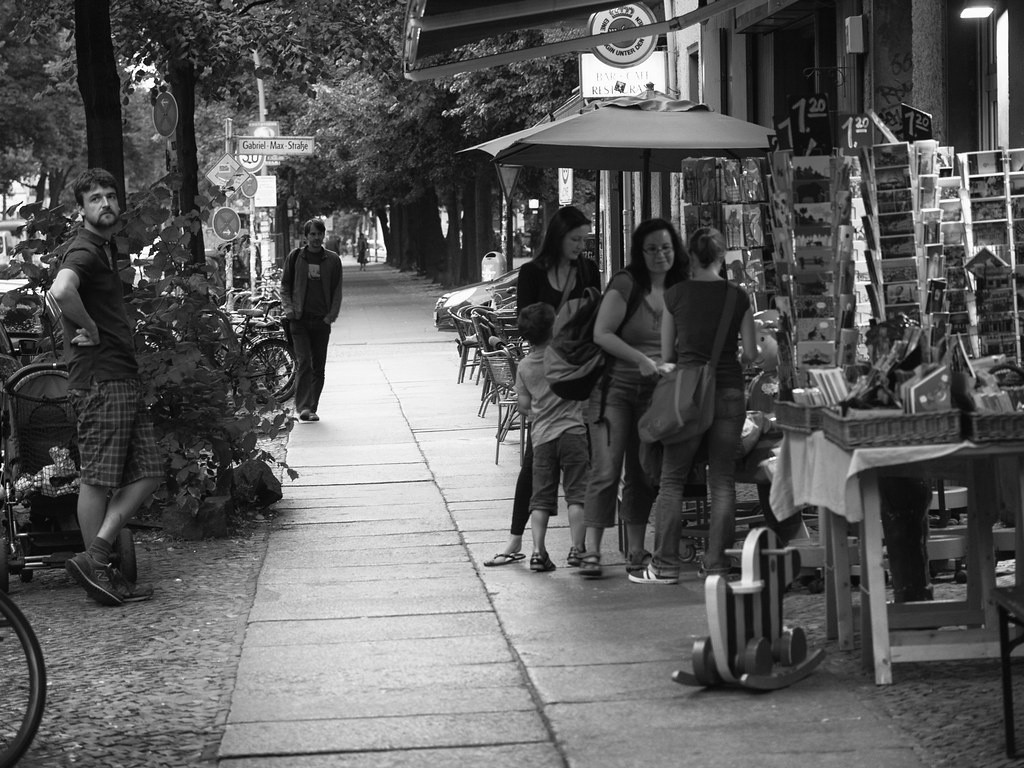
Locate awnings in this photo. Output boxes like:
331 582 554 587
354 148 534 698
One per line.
399 0 750 82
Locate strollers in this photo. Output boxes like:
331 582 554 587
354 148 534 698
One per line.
0 315 140 592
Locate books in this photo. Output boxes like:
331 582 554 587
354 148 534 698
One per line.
766 95 851 409
678 156 763 300
963 150 1024 414
858 103 977 380
898 364 952 414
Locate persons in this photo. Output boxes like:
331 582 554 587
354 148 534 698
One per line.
49 169 164 606
627 227 761 584
281 220 342 423
358 234 370 269
578 217 690 575
482 205 599 568
515 302 590 571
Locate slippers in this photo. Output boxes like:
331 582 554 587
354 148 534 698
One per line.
483 553 524 566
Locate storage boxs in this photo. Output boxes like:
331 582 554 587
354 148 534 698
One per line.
772 394 1024 448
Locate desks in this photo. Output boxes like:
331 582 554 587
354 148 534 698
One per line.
759 429 1024 684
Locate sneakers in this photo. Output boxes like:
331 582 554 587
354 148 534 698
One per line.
697 566 730 581
110 567 153 602
628 564 678 585
65 551 123 606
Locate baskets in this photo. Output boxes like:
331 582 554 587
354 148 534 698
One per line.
774 391 1024 453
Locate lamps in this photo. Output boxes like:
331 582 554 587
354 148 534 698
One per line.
960 0 995 20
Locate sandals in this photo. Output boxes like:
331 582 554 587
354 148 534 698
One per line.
300 407 319 421
626 556 653 573
578 552 602 575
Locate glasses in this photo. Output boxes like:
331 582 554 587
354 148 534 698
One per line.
642 246 671 257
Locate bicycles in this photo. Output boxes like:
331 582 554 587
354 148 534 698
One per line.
202 234 301 405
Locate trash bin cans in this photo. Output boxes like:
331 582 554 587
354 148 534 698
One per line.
480 251 507 282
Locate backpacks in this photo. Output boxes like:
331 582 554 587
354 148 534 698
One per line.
542 270 638 402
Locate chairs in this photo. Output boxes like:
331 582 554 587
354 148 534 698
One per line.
988 584 1023 734
447 296 529 465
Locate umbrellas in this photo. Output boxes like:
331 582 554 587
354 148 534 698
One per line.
453 80 776 265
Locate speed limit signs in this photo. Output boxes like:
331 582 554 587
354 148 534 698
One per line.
237 154 266 175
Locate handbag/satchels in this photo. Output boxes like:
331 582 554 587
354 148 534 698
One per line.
637 362 717 446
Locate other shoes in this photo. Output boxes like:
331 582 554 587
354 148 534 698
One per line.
567 548 580 564
530 553 554 571
